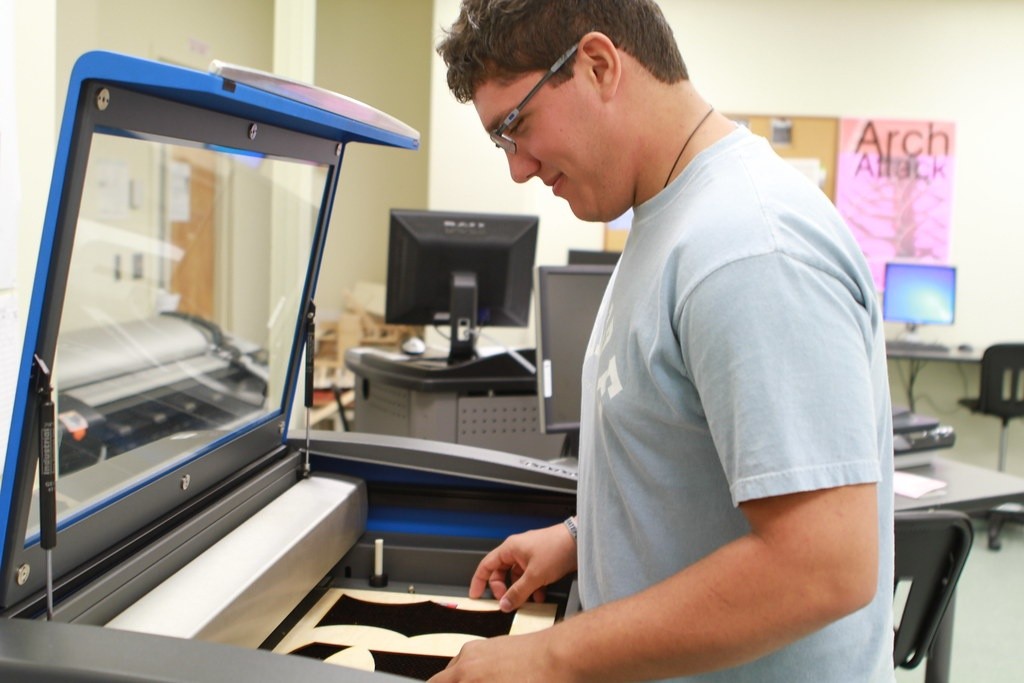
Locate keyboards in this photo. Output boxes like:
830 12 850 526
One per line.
885 342 949 352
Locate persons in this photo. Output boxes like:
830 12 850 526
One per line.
429 1 894 683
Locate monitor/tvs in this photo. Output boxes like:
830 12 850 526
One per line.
385 209 626 458
882 264 956 327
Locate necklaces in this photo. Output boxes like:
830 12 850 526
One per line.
664 108 715 188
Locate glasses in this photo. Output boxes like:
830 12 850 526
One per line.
490 34 618 153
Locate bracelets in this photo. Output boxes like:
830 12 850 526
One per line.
565 516 577 541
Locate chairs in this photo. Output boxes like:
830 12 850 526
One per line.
894 510 974 682
943 342 1023 550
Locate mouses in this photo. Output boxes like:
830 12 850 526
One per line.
400 335 426 355
958 345 974 353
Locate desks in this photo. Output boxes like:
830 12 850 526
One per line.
888 345 983 408
893 458 1023 683
